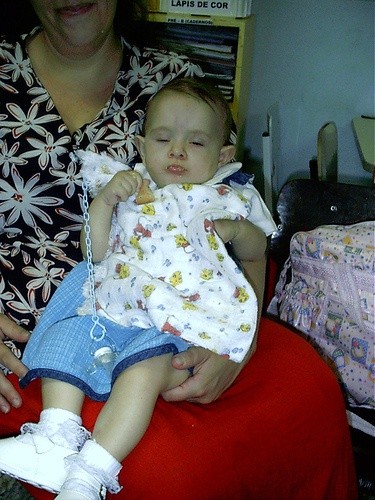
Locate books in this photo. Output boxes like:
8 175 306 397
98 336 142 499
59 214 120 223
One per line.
150 21 239 104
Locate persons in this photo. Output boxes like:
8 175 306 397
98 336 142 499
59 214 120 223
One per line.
0 75 278 500
0 0 358 500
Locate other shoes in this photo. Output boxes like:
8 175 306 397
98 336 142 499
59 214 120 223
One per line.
49 470 105 499
0 431 72 491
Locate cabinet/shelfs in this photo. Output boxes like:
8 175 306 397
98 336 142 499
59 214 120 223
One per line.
143 13 256 166
